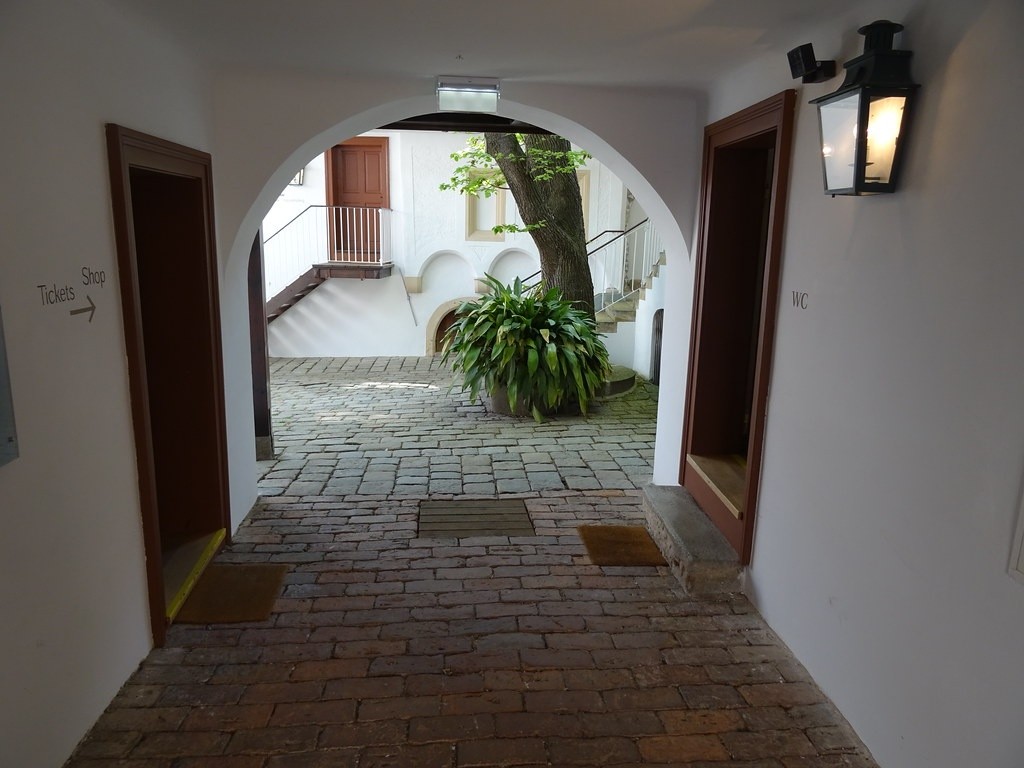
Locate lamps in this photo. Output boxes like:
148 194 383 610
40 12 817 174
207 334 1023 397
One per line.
808 19 921 199
287 168 303 186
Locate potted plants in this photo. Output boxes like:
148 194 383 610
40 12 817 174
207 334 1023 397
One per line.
439 272 614 424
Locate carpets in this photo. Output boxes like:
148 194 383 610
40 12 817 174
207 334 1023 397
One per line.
577 524 672 566
173 562 291 625
